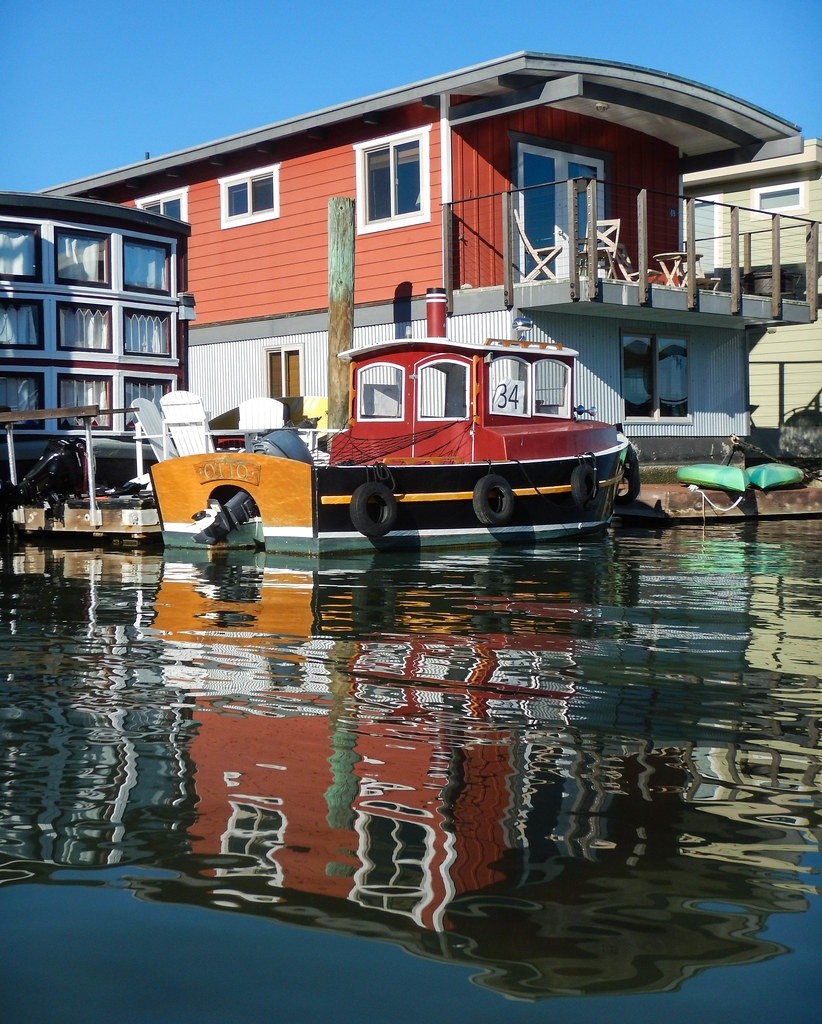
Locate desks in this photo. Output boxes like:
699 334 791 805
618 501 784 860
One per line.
653 252 703 287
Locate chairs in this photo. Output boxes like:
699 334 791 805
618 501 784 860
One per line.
579 219 620 279
514 209 562 283
616 243 662 284
675 260 721 291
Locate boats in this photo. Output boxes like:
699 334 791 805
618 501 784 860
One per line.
152 563 622 931
131 288 629 556
